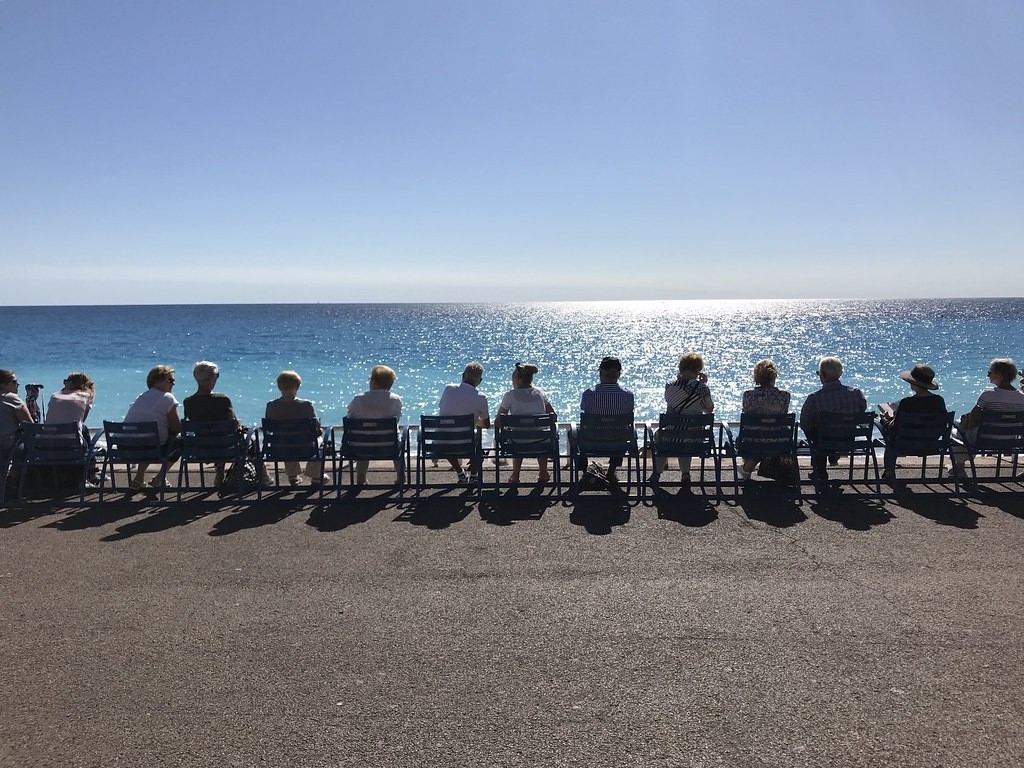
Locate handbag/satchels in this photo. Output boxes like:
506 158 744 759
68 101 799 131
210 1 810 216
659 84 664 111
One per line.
580 461 610 489
488 428 508 466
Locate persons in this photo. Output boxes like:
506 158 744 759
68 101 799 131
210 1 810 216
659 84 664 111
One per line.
800 358 867 480
879 364 950 479
736 359 791 480
112 365 181 489
431 362 490 484
347 365 406 484
184 361 274 486
0 369 36 484
945 358 1024 476
265 370 331 485
494 363 558 481
40 372 111 489
568 357 635 481
646 352 714 488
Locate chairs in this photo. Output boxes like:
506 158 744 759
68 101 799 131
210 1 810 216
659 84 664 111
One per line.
17 421 105 507
177 419 260 503
258 418 337 504
416 416 484 496
99 420 189 504
0 434 23 507
952 409 1024 485
794 410 881 498
337 417 411 503
569 413 641 497
644 412 720 505
871 411 959 493
494 414 562 495
718 412 803 500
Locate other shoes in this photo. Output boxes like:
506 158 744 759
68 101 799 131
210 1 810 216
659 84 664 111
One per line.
946 464 966 477
359 479 370 486
607 473 619 480
538 472 550 482
311 474 330 485
809 471 828 479
289 475 303 485
882 469 895 479
829 459 838 465
736 464 752 480
458 474 469 485
509 476 520 483
85 468 273 488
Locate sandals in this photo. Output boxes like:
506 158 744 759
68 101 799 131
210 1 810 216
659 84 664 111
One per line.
647 473 661 483
681 472 691 489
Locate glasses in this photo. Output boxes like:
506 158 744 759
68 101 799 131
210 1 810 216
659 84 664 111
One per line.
210 373 220 378
815 370 821 377
7 379 17 385
163 377 174 384
596 368 601 374
367 375 372 382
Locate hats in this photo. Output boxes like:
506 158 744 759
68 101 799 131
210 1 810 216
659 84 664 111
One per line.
901 364 938 390
